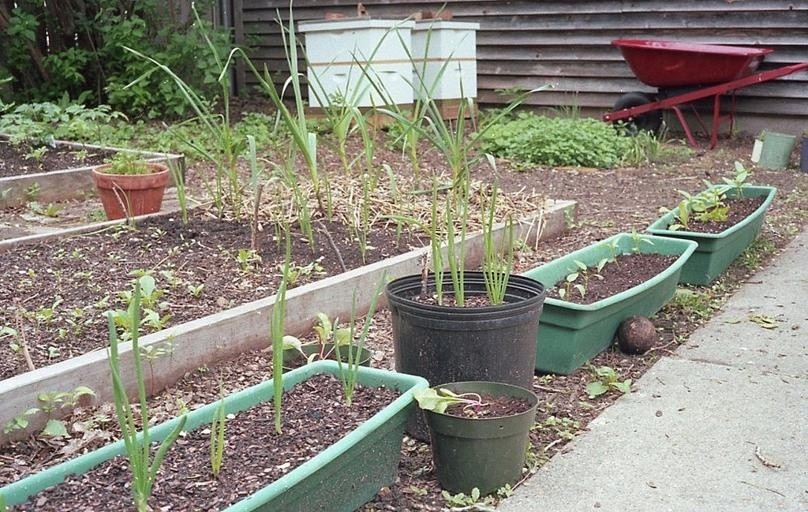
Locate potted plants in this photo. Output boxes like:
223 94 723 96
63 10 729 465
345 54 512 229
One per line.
408 376 537 495
92 147 172 220
646 156 779 291
377 143 551 447
0 221 428 512
522 227 700 379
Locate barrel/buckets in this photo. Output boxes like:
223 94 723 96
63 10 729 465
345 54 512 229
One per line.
422 379 540 498
385 270 548 445
751 129 807 174
279 343 374 376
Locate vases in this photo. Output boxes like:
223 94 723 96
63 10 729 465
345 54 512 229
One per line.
748 127 794 174
797 131 807 174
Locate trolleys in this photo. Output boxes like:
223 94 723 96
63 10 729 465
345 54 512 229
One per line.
601 34 808 148
